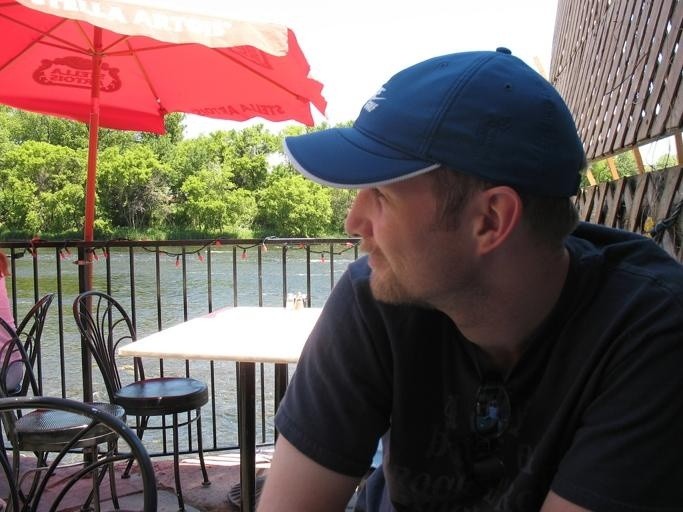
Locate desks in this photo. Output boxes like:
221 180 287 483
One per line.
119 303 323 510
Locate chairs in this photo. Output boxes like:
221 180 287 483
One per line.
0 313 126 504
71 289 210 486
1 395 160 510
0 293 58 396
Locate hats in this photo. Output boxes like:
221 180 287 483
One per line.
284 48 586 198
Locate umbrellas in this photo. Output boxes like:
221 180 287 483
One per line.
0 0 326 472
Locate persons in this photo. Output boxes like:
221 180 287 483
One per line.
0 251 23 393
255 48 683 512
